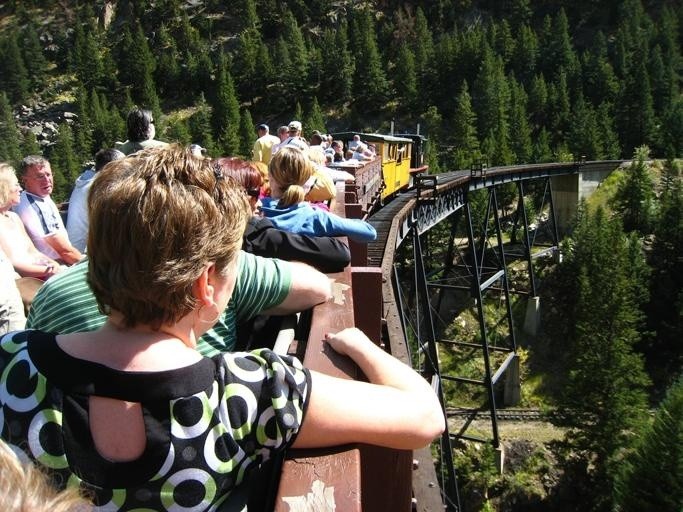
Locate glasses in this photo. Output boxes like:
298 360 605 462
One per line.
28 172 55 180
149 118 155 125
248 185 260 202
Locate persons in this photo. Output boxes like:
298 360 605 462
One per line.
279 119 310 159
308 135 326 164
12 153 86 271
307 145 357 186
323 133 376 163
214 154 354 274
67 144 130 258
277 125 290 146
120 107 168 156
26 251 331 355
0 143 448 512
259 147 379 250
0 158 70 285
252 122 283 163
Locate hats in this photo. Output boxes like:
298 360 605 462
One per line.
288 121 302 131
